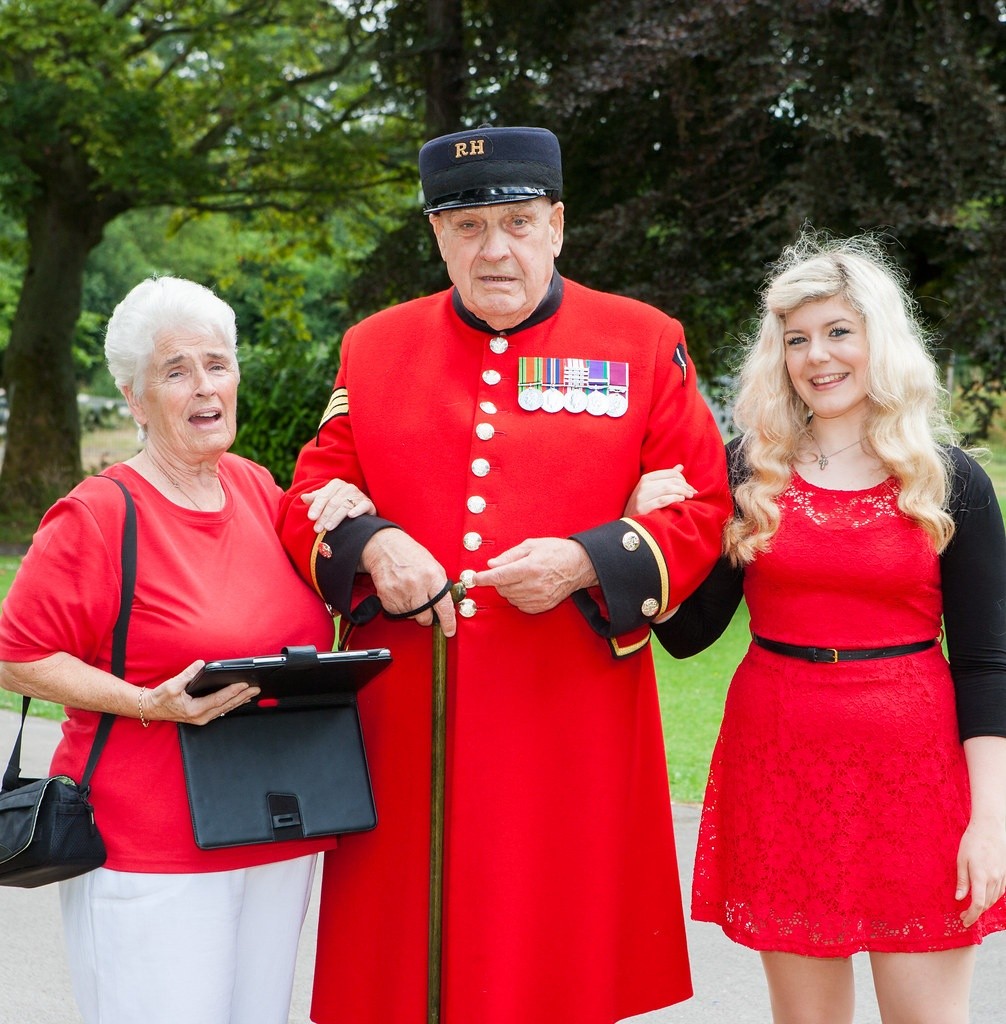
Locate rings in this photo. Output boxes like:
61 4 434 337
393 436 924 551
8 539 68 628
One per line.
345 498 356 507
221 713 225 716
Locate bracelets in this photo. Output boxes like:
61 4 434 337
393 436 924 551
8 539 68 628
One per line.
139 686 150 727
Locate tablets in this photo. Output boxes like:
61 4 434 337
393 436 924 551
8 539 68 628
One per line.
187 648 391 720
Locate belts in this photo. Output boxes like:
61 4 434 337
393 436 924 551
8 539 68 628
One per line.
752 632 936 663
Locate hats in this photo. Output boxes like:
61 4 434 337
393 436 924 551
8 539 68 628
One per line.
420 127 563 215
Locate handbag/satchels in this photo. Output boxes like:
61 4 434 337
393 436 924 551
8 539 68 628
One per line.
0 775 106 888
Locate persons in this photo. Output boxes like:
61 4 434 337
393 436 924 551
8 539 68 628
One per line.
624 252 1005 1024
277 126 734 1024
0 276 375 1024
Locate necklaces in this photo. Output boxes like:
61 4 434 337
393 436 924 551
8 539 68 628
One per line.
172 480 222 511
809 417 867 470
